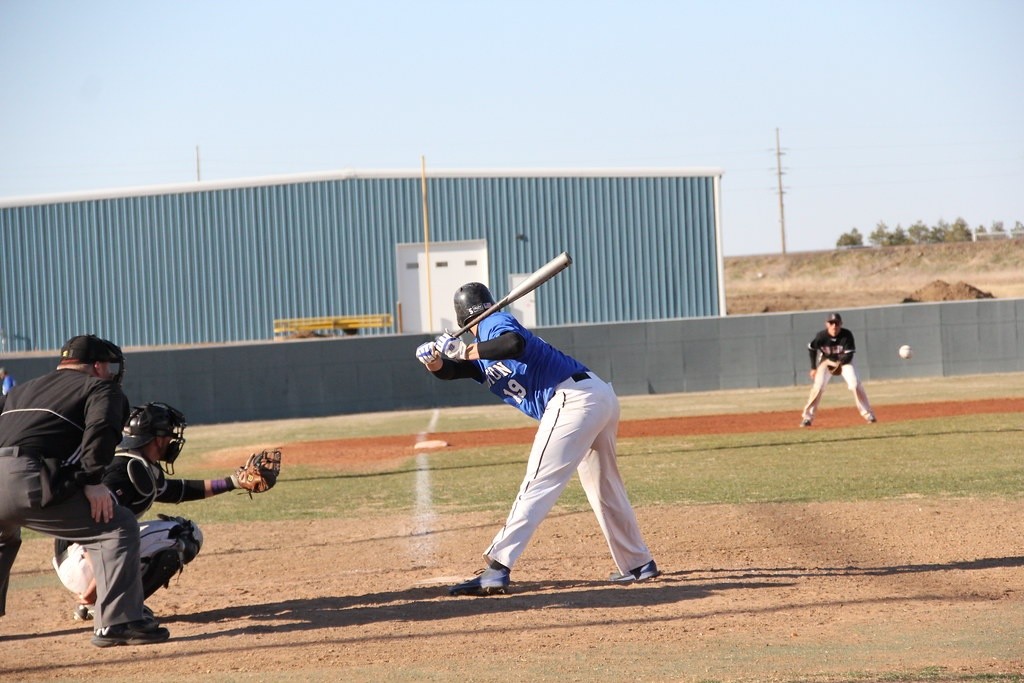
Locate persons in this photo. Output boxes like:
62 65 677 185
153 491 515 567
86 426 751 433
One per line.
51 402 283 622
802 312 876 425
416 281 662 596
0 366 19 395
0 334 170 646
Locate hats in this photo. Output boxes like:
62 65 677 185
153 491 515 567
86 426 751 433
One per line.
827 313 842 323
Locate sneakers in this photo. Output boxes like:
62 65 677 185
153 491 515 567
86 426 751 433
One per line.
91 622 170 647
609 560 661 582
449 568 511 595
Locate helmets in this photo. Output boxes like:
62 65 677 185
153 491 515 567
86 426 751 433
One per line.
61 334 126 363
116 401 183 450
454 282 500 334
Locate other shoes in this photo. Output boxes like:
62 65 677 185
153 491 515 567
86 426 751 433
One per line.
868 416 877 424
800 419 811 428
74 603 154 622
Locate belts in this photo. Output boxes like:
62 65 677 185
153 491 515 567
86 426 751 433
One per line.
0 448 37 457
552 372 592 397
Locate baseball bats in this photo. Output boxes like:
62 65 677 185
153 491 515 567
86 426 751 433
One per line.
434 251 573 350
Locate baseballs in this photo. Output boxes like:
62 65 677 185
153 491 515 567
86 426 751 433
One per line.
899 345 913 360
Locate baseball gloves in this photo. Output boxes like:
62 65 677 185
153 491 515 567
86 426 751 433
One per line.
827 363 842 376
230 446 284 499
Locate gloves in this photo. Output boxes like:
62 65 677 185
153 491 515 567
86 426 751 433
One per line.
416 342 439 366
434 328 467 360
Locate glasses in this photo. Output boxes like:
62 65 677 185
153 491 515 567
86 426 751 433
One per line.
830 321 839 325
